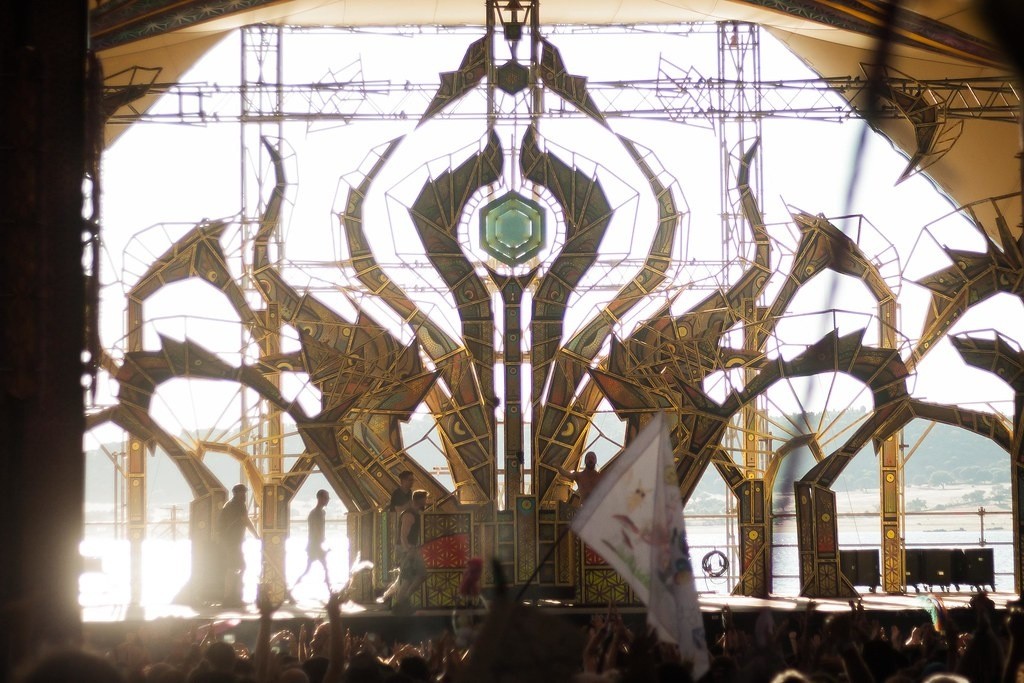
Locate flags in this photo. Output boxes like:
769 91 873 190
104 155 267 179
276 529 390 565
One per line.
571 410 709 683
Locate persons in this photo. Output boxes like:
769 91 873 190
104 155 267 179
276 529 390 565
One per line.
557 452 600 504
1 471 1024 683
